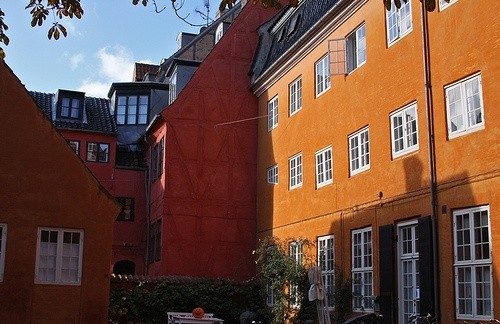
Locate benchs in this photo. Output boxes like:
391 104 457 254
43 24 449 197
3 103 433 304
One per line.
167 311 213 324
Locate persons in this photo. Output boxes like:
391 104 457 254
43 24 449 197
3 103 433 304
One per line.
239 302 260 324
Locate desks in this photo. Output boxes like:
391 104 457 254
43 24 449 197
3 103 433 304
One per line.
173 316 224 324
